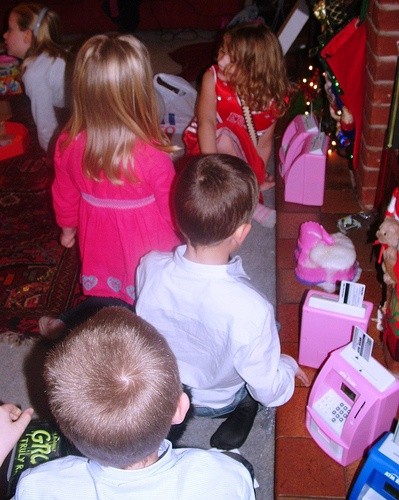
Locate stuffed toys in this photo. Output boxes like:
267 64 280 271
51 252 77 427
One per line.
376 217 399 284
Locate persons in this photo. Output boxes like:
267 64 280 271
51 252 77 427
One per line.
183 24 292 230
136 154 308 489
4 5 73 154
51 33 169 307
0 307 255 500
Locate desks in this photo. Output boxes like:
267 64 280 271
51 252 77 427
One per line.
274 136 387 500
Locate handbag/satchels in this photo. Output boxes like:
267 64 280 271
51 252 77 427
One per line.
153 73 199 158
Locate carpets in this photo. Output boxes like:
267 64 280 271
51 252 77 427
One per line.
0 106 86 347
